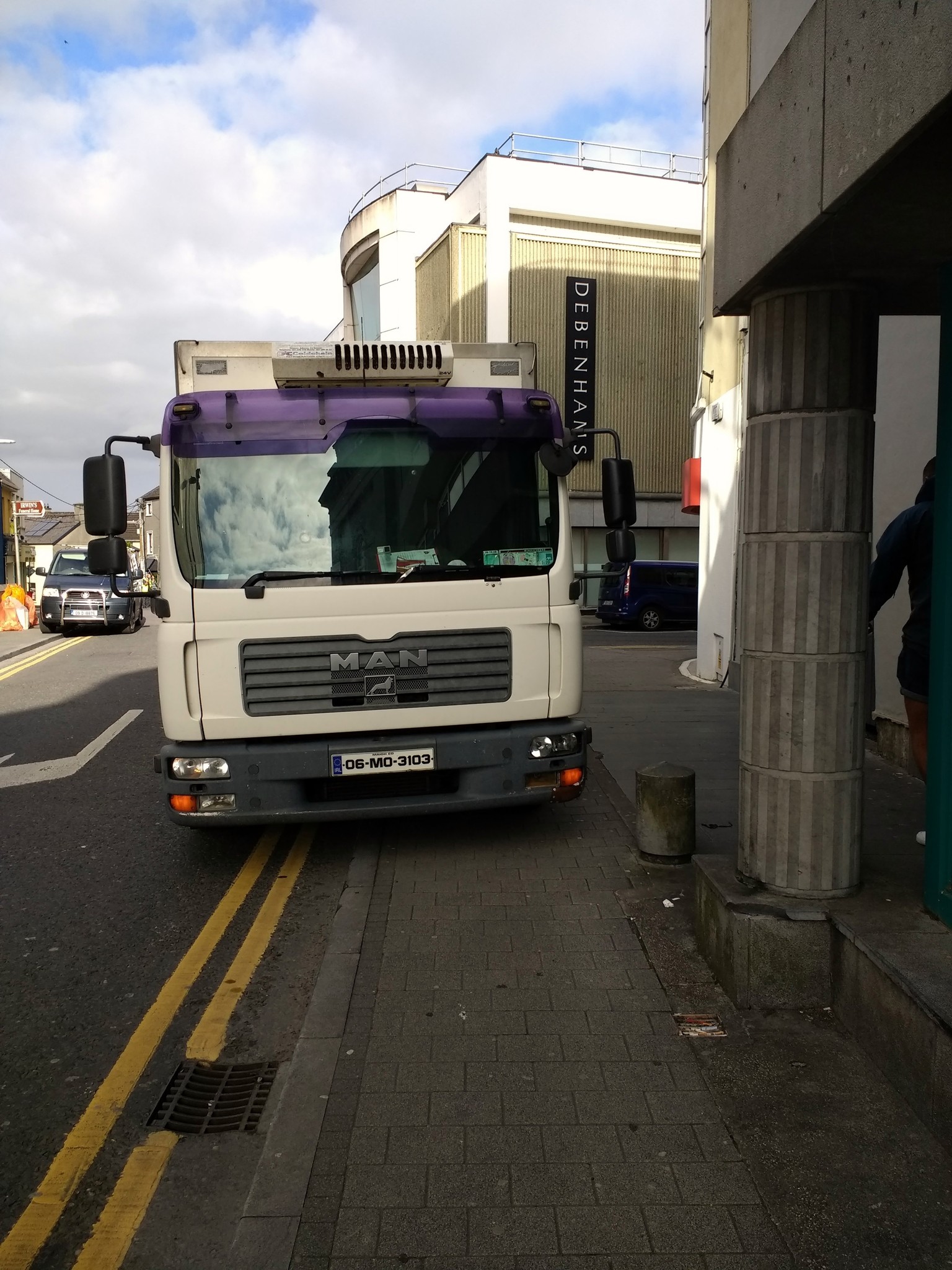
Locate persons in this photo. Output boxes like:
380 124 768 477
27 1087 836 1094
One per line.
869 457 936 846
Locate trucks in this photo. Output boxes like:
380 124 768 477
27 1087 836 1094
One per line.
82 339 637 828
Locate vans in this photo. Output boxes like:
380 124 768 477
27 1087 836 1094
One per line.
596 560 699 631
35 549 144 633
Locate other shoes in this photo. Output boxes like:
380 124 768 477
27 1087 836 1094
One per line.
916 831 926 845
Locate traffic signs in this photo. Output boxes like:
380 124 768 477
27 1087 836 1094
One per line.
13 500 46 517
